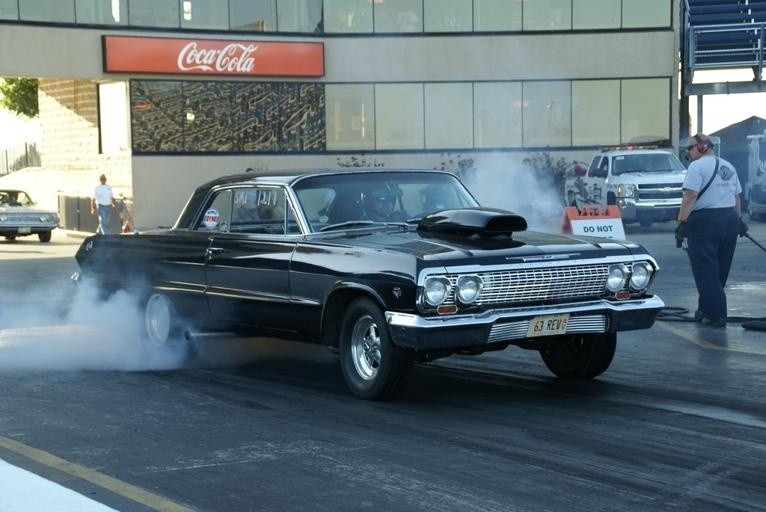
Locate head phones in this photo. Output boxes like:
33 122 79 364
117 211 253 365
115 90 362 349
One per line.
694 134 710 153
352 178 367 218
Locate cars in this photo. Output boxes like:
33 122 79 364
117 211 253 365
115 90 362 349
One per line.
0 189 60 243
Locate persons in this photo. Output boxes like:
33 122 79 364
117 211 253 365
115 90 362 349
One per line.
675 134 749 327
364 185 411 224
238 168 260 222
91 174 117 234
9 194 22 205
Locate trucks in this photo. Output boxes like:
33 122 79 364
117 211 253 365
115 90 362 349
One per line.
745 129 765 220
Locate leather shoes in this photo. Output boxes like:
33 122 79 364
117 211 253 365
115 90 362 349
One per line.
695 309 727 327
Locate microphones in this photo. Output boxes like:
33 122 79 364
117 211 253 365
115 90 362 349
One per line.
685 155 689 160
363 205 390 219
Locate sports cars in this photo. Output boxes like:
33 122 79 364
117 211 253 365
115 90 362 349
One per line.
74 168 664 403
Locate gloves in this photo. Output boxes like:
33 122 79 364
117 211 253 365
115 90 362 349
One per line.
675 222 685 249
737 216 748 237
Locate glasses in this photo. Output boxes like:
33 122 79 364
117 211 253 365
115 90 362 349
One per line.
376 197 392 202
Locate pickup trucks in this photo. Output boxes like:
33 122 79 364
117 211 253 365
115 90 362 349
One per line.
564 143 689 227
